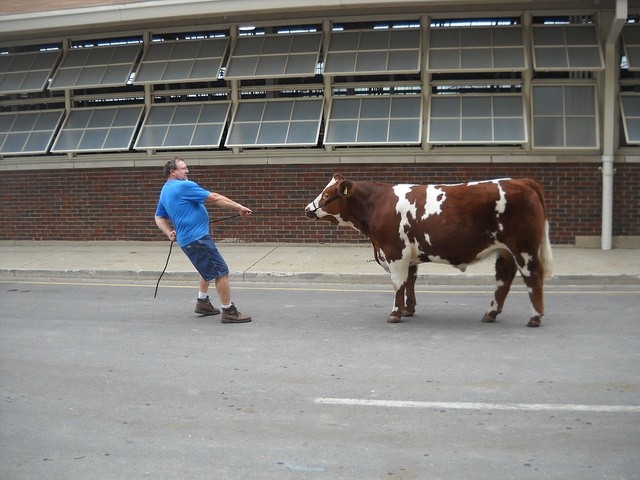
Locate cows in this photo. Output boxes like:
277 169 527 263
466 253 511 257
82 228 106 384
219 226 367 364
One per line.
301 170 556 328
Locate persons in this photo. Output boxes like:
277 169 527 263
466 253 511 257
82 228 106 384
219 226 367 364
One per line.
154 157 252 323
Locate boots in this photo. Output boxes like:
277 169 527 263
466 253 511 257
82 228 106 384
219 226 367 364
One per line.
195 295 220 315
221 301 251 323
